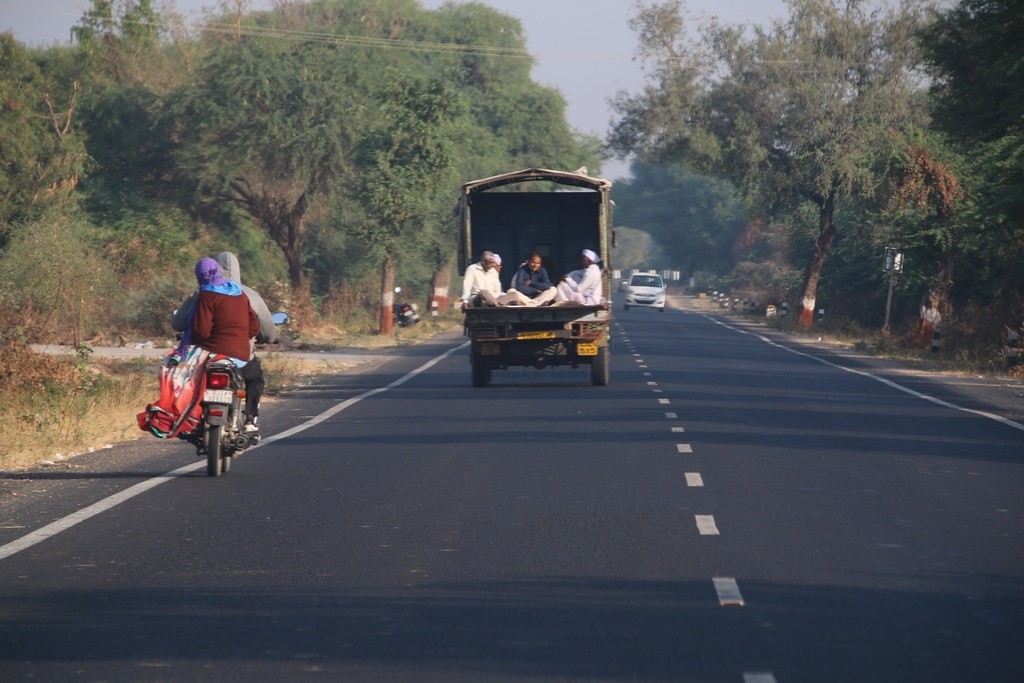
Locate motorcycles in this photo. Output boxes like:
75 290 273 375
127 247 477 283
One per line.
393 287 418 327
170 308 289 477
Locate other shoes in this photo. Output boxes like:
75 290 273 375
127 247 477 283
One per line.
243 422 259 433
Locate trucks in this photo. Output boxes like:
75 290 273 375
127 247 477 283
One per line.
460 168 618 387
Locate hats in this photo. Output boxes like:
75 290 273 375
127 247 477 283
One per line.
493 254 502 265
582 248 600 263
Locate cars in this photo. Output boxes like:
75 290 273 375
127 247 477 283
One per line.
623 271 667 312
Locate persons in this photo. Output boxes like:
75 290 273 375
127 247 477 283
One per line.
136 250 277 439
462 249 603 310
